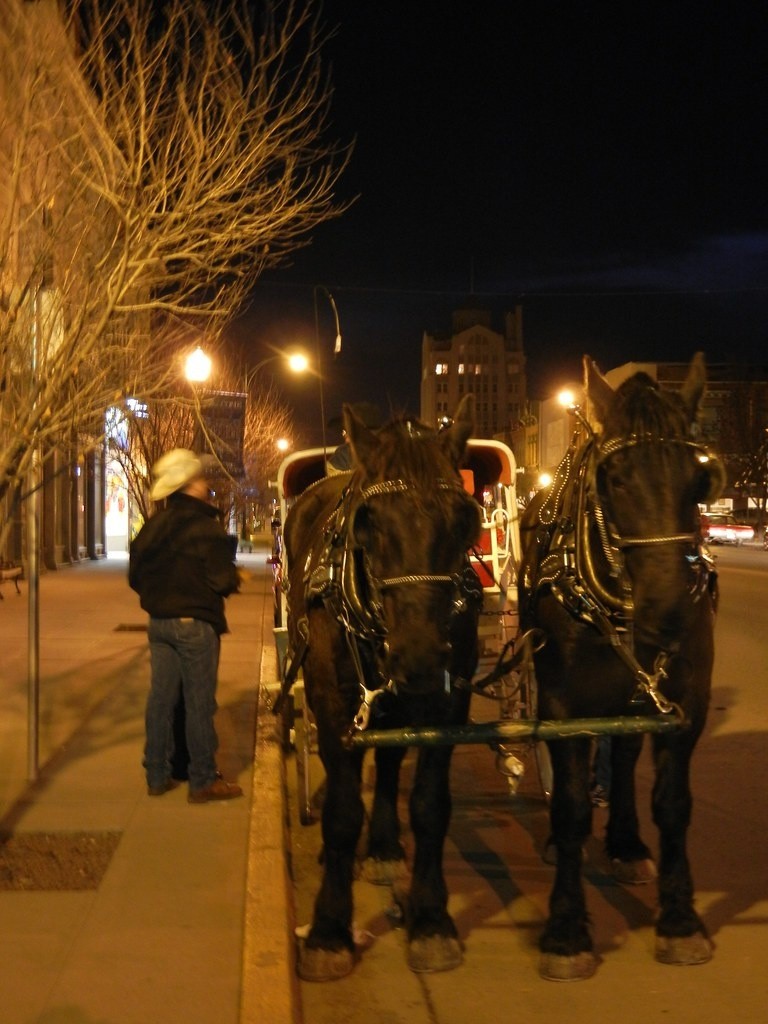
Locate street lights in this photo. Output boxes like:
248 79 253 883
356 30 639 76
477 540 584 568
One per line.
242 355 306 541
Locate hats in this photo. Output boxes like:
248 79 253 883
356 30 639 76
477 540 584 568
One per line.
149 449 206 501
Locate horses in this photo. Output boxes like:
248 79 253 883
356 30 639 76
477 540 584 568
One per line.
283 390 487 990
516 347 729 983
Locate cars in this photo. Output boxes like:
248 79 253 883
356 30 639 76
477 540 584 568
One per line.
700 512 754 546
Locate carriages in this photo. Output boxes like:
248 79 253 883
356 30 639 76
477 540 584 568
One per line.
266 351 725 982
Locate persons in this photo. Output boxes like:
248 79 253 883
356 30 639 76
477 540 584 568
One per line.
127 448 242 804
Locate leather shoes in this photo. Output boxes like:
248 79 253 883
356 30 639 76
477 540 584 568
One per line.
147 776 181 795
188 778 243 802
